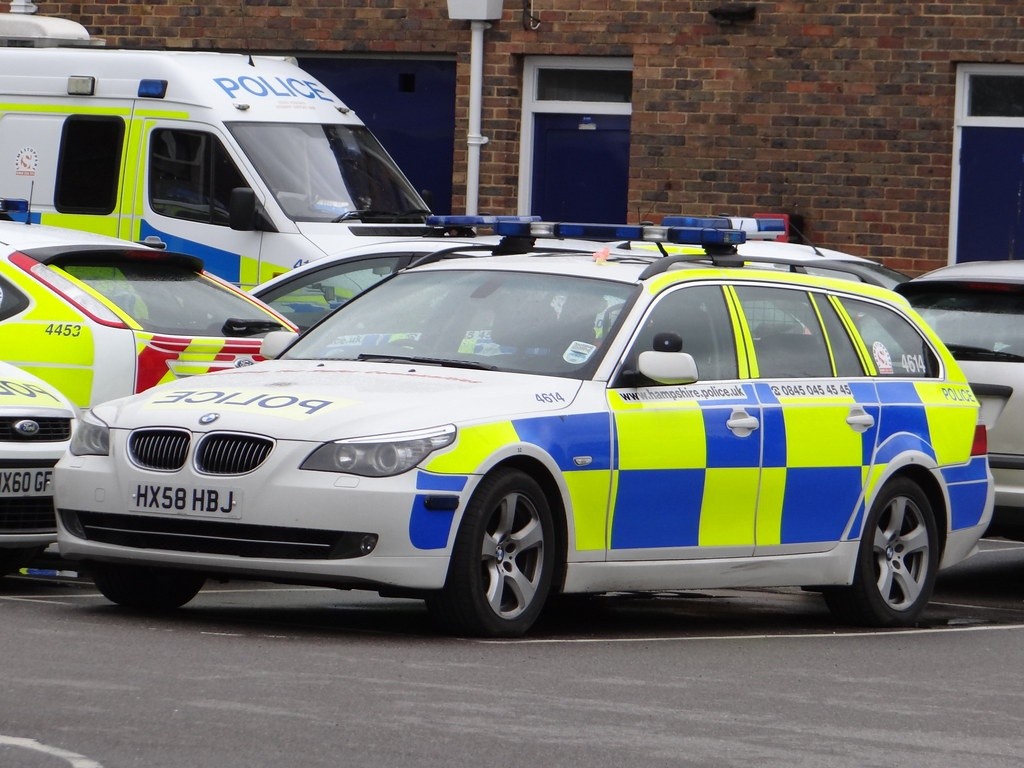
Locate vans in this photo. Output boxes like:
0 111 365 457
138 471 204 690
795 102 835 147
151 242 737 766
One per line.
1 11 514 362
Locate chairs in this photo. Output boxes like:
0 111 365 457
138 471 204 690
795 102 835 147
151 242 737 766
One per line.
600 298 723 380
478 298 557 374
749 323 865 381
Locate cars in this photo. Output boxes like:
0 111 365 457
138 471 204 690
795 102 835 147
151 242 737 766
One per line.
1 220 299 421
1 360 80 578
605 215 914 335
243 238 504 363
858 260 1023 542
52 220 995 638
